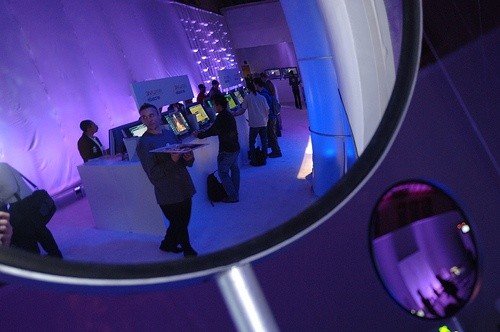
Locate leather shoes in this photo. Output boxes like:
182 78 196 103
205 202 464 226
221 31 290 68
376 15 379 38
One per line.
160 242 182 253
185 248 197 255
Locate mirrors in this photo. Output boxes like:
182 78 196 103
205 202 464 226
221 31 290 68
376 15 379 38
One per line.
369 179 480 321
0 0 423 289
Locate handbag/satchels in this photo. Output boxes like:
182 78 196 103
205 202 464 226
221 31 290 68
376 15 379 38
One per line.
248 147 261 160
7 171 56 239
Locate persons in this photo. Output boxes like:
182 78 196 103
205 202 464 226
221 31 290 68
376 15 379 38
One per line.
0 60 302 258
417 248 474 317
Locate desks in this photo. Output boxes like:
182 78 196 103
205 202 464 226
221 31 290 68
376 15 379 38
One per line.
77 108 250 236
424 290 456 319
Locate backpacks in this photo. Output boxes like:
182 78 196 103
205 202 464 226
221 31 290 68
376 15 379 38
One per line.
207 170 226 207
262 90 281 116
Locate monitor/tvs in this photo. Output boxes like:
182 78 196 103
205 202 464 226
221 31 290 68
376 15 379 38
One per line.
282 67 299 78
109 82 250 160
268 68 282 79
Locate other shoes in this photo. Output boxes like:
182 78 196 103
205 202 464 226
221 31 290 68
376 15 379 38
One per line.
223 197 237 202
269 152 282 157
250 160 266 165
